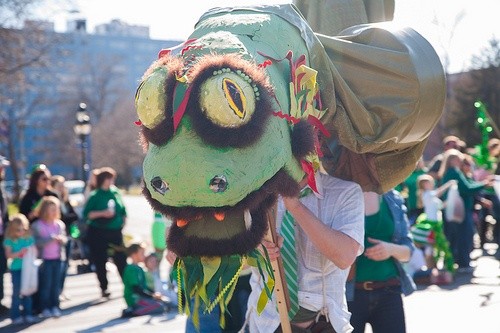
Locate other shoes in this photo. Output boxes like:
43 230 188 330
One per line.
61 293 70 300
103 289 110 297
59 295 65 302
25 316 34 323
53 307 60 317
12 318 23 324
122 307 134 318
43 308 52 318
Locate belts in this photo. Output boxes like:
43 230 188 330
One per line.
355 279 401 290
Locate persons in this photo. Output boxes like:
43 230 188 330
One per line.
0 154 77 324
394 135 500 270
261 171 364 333
123 244 166 317
346 188 418 333
81 167 128 296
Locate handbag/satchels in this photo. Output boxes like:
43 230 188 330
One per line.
446 185 464 222
20 245 38 296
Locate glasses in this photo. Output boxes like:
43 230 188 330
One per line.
38 177 49 183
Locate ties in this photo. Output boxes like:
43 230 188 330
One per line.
278 188 314 323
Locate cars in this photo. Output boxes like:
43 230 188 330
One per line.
63 179 85 217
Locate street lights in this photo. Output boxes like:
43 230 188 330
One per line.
431 11 469 152
73 102 92 182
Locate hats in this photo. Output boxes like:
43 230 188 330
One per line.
488 138 500 149
417 174 433 188
443 136 459 147
